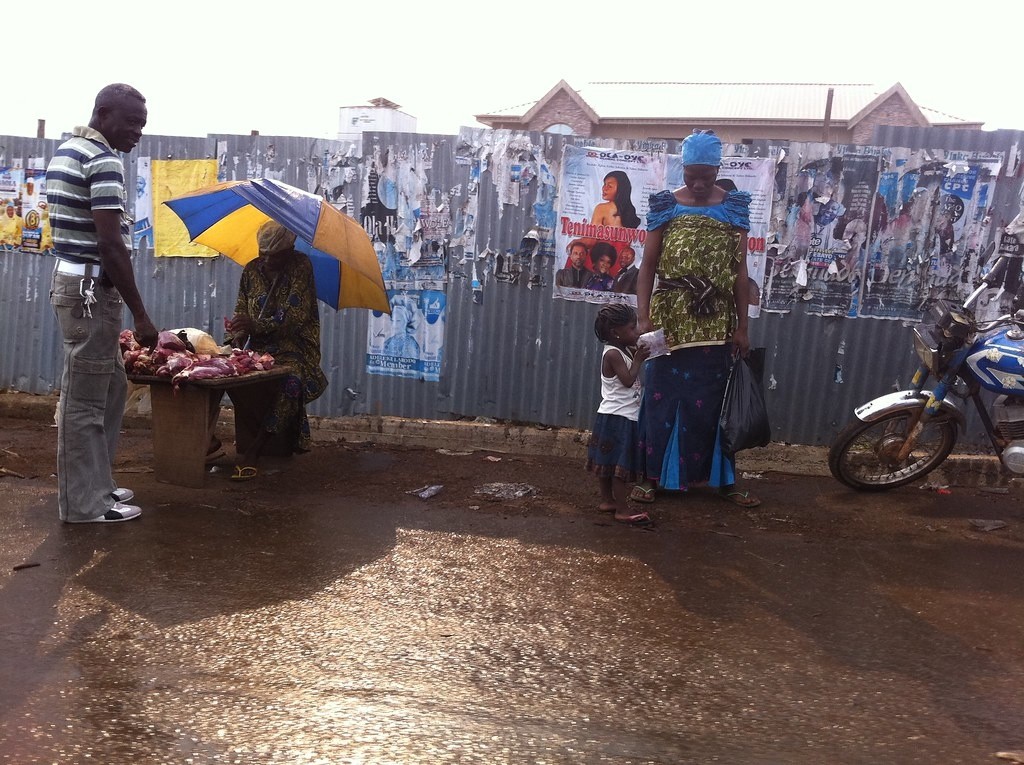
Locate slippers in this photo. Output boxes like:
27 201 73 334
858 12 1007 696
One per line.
629 483 657 504
718 490 761 507
230 466 258 480
203 445 226 461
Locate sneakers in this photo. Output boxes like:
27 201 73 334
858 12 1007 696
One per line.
107 488 133 504
67 504 142 525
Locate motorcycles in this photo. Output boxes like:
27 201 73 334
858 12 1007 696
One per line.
827 239 1024 496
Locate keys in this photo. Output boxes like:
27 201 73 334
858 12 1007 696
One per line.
78 276 98 319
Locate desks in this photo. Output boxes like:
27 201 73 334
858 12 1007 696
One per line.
125 364 294 489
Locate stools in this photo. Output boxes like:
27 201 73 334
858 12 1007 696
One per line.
233 382 295 456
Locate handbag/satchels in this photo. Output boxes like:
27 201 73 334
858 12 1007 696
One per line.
721 344 770 456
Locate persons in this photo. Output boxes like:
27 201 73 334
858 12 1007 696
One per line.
554 170 643 299
223 219 329 457
42 83 161 523
836 218 868 273
629 127 763 507
584 304 650 522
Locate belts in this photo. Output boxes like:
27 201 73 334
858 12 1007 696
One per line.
49 259 100 281
